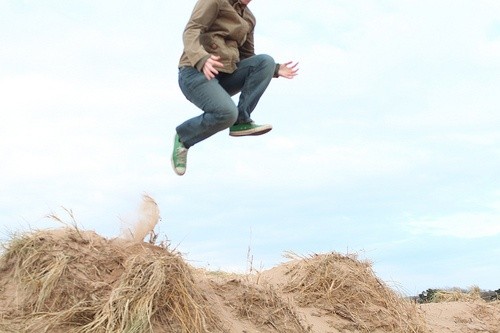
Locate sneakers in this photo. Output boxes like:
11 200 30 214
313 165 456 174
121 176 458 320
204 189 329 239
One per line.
172 132 188 176
230 123 272 137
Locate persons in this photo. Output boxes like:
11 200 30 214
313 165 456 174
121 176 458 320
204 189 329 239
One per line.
171 0 299 175
